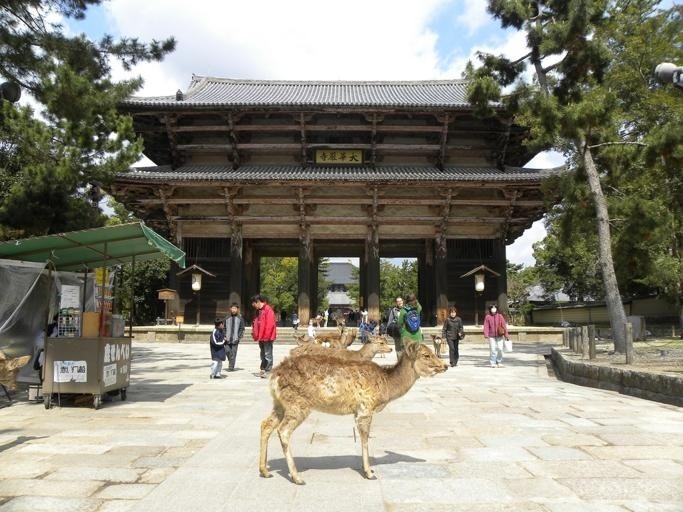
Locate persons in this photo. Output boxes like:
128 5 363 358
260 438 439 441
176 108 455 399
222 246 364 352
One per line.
349 306 374 342
307 308 329 339
441 306 464 366
482 303 509 368
385 292 423 353
32 309 78 406
291 311 299 331
208 303 245 381
250 294 276 379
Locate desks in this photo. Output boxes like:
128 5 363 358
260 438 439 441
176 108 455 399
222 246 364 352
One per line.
41 335 134 407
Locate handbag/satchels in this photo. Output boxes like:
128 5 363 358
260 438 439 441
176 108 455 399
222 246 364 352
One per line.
459 329 465 339
498 326 504 336
388 318 399 338
504 341 513 353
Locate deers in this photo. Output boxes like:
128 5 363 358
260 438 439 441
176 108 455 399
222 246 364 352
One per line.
0 353 30 397
259 315 450 485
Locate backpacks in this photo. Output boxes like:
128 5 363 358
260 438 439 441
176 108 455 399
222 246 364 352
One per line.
404 310 420 333
